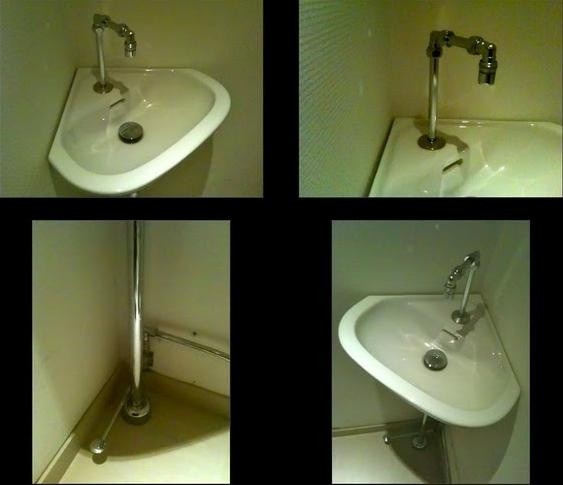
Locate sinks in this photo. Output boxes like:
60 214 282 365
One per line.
338 294 521 427
47 65 232 197
368 116 562 198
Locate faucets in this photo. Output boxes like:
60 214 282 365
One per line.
91 13 138 95
418 29 498 150
443 249 481 324
